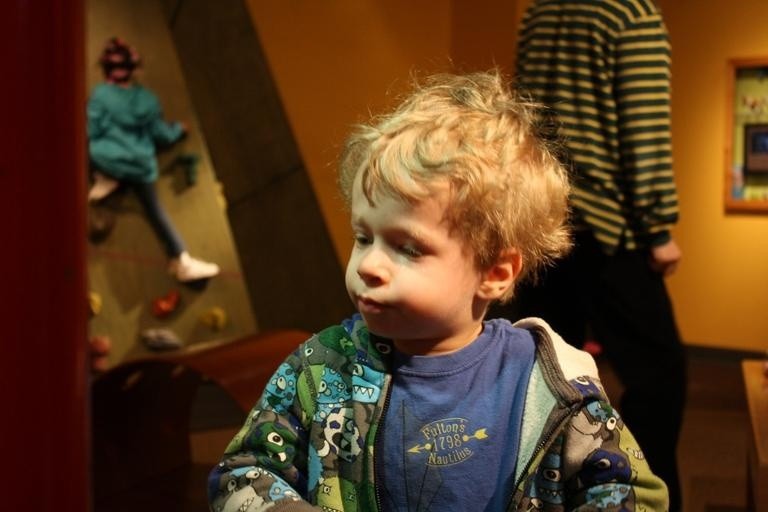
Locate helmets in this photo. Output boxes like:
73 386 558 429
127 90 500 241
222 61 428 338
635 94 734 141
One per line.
100 38 141 82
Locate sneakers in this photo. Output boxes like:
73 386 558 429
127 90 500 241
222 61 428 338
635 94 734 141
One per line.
172 250 221 282
87 178 119 202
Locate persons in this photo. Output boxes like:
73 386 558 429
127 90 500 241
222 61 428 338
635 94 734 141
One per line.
85 36 221 286
203 63 670 512
491 2 690 512
